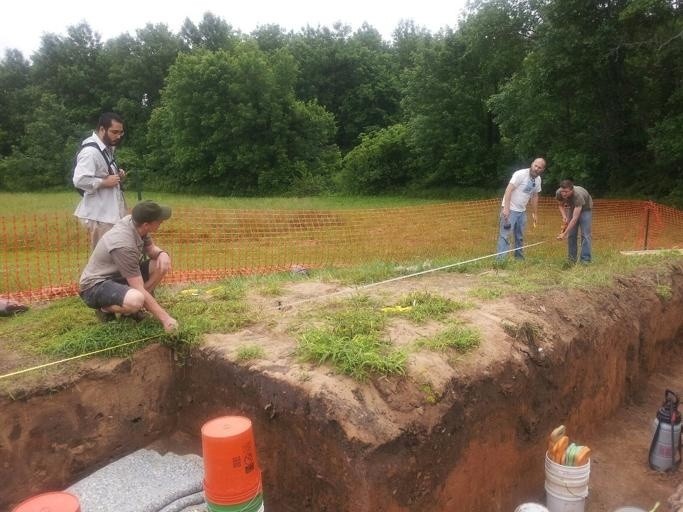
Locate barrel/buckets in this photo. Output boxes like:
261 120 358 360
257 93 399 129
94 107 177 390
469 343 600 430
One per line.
199 415 264 512
12 491 81 512
543 449 591 512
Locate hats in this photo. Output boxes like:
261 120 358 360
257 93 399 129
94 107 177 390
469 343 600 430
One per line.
132 200 171 222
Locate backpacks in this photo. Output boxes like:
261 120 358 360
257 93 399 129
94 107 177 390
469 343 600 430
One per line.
73 143 114 197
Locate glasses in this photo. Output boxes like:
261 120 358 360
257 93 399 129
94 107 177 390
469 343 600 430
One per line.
532 177 536 188
110 129 124 136
561 191 570 193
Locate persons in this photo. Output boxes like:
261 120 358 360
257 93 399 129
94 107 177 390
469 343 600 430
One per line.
72 111 124 253
78 199 181 333
555 179 594 264
495 157 547 263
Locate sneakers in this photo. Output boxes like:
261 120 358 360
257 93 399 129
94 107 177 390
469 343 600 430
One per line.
121 310 145 321
95 308 116 322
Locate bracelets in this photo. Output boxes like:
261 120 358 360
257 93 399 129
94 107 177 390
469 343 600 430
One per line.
157 251 169 257
